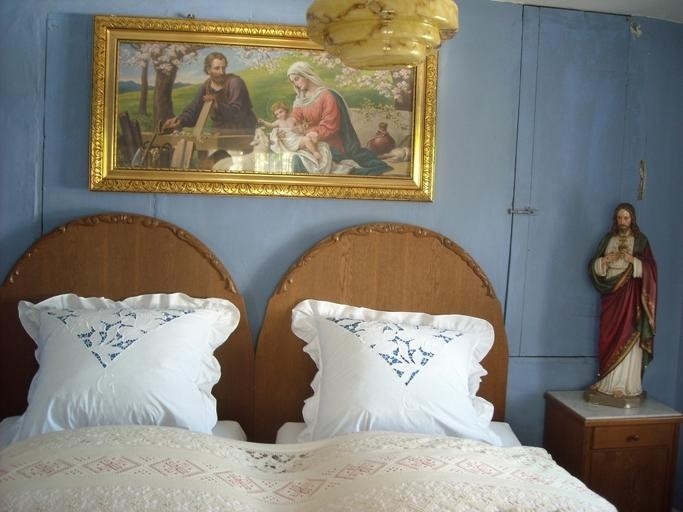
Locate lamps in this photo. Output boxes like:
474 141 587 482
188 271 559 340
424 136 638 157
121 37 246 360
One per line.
306 0 459 70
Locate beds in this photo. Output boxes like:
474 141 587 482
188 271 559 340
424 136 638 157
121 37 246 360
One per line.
2 213 629 512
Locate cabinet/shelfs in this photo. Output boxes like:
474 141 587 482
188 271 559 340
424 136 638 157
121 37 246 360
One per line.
505 5 648 359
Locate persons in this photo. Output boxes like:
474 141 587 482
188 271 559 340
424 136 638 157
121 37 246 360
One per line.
588 202 658 397
257 101 321 160
161 53 256 170
254 61 394 180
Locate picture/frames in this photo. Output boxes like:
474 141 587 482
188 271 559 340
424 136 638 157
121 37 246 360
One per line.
89 6 439 202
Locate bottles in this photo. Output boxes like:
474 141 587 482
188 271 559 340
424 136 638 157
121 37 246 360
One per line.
369 123 396 155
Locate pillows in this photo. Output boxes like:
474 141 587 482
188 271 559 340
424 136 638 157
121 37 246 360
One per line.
276 297 501 448
15 292 239 439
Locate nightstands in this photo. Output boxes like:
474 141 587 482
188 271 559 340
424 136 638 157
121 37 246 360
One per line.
542 390 682 510
